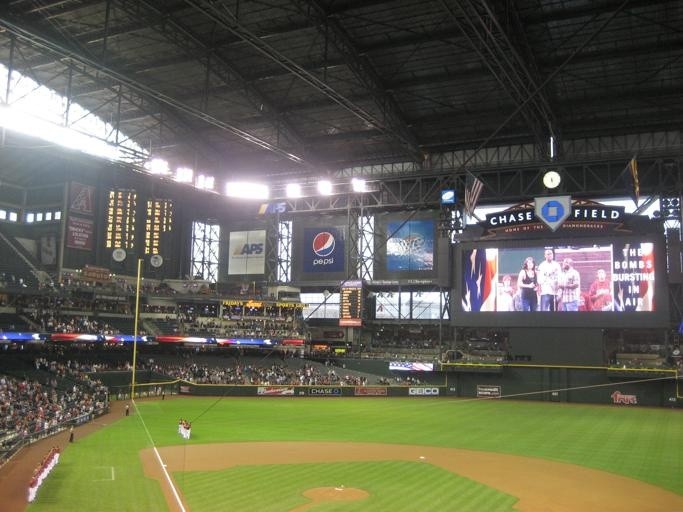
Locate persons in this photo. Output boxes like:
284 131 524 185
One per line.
499 247 611 311
28 445 60 501
178 418 191 439
0 272 303 386
116 387 165 400
0 385 108 464
466 247 483 312
126 404 130 416
301 325 438 386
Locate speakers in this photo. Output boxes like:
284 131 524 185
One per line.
667 227 681 284
437 238 451 288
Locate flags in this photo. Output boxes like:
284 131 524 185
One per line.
43 237 54 257
464 171 484 217
628 159 641 208
